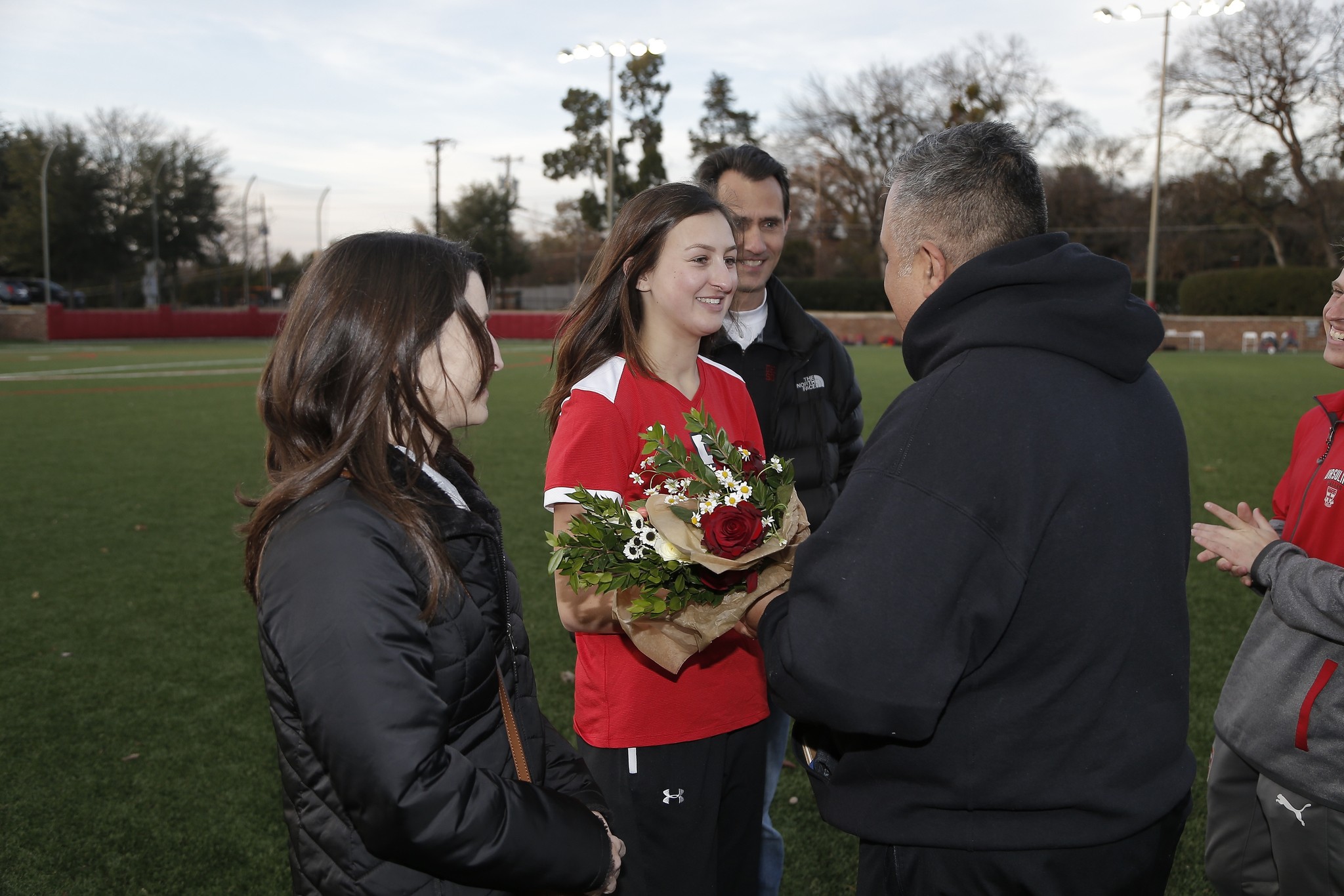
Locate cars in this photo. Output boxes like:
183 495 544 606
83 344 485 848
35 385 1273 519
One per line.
1 276 85 308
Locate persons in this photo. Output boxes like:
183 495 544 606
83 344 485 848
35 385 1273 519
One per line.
735 118 1196 896
1181 252 1344 896
535 179 809 896
234 227 634 895
684 142 870 534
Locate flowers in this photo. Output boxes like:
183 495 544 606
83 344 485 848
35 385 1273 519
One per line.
544 396 812 675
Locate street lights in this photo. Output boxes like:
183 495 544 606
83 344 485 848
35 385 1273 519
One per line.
554 36 668 234
425 137 453 240
1090 0 1245 309
493 152 527 307
